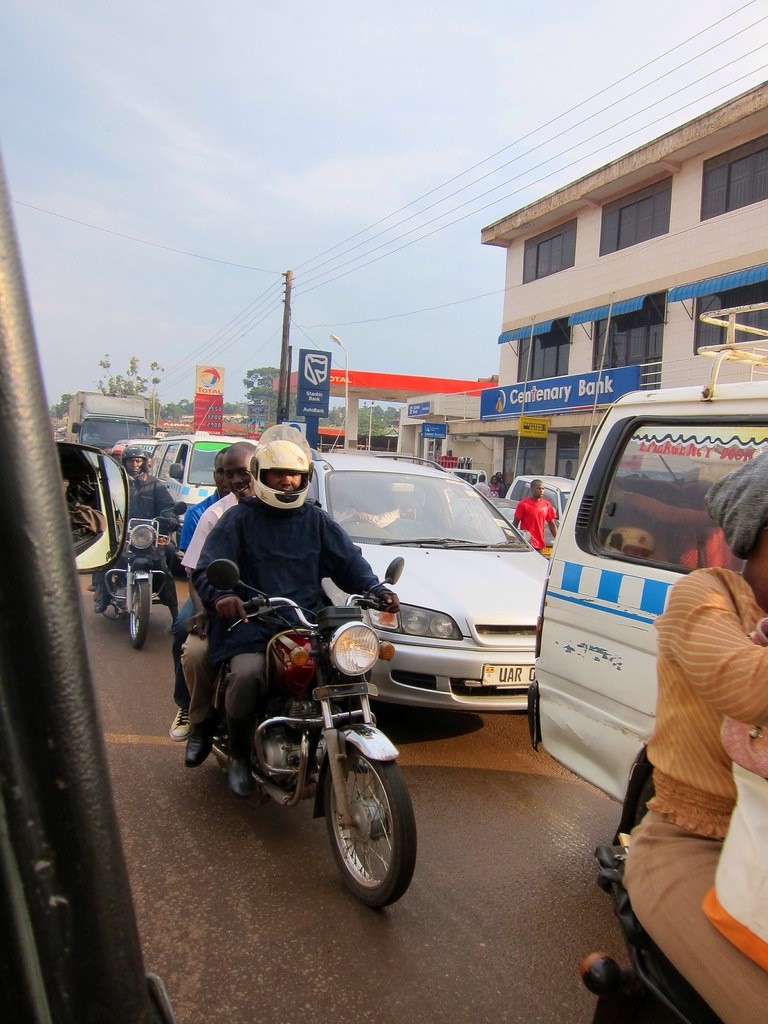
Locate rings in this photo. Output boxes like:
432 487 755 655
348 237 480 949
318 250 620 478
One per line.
193 625 197 629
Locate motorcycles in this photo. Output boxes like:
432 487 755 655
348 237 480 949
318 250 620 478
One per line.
98 500 189 650
169 553 419 909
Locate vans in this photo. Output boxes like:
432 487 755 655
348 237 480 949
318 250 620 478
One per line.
107 434 261 574
525 300 768 820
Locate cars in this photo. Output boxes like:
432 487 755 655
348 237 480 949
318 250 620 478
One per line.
440 467 578 562
305 446 553 715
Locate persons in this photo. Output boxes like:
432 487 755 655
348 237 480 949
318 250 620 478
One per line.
512 479 558 554
191 425 400 799
495 472 506 498
487 476 499 498
169 447 232 742
180 442 257 768
473 475 490 498
86 446 184 623
621 450 768 1024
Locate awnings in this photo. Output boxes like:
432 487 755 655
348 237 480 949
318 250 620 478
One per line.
568 289 667 341
667 263 768 320
498 314 573 356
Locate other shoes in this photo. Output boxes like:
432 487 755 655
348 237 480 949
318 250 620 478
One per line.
93 584 101 602
87 584 96 591
94 594 111 613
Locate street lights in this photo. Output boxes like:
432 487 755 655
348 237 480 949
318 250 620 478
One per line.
330 333 351 451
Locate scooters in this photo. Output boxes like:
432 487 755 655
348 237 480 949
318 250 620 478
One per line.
577 737 768 1024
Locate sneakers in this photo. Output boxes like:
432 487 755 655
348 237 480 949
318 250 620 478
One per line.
169 707 193 741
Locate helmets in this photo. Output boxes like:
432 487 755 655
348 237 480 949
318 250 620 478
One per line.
111 445 125 454
250 439 314 510
122 445 148 470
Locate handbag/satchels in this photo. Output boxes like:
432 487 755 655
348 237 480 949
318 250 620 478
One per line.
719 617 768 779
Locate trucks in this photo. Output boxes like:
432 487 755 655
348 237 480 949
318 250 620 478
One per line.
65 390 165 451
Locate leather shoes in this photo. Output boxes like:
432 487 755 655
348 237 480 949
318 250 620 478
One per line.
185 709 222 767
227 756 254 798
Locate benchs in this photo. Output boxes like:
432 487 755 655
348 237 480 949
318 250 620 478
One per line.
605 475 742 573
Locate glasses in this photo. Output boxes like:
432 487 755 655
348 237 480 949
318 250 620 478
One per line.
224 468 251 479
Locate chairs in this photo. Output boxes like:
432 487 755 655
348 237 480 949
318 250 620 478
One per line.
66 475 94 504
392 485 427 536
